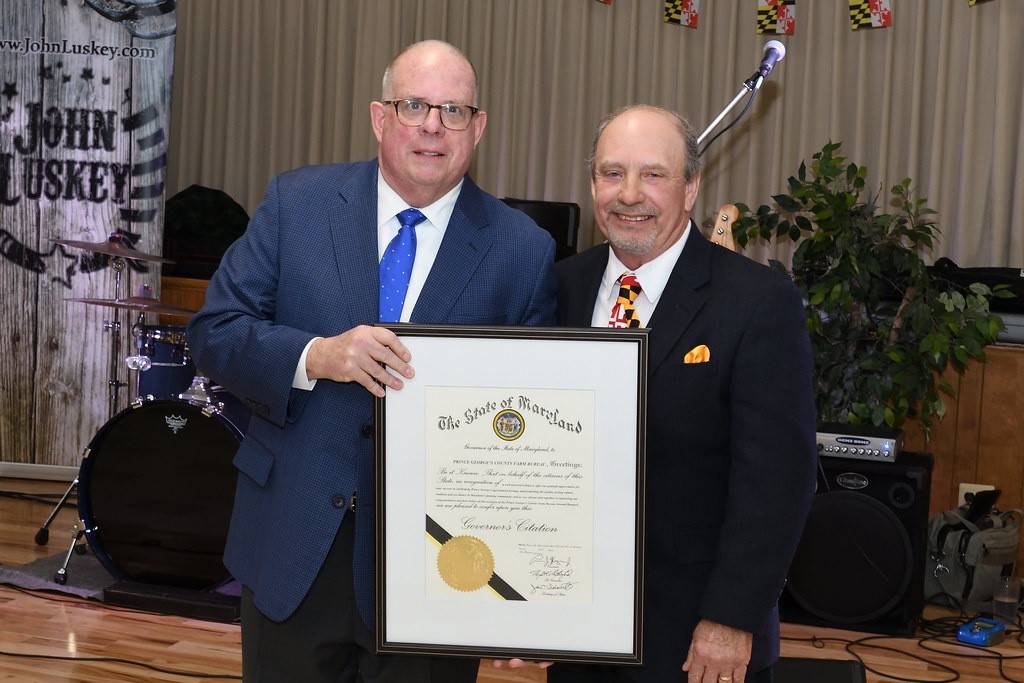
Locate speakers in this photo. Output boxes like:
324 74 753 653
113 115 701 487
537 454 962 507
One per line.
778 452 934 638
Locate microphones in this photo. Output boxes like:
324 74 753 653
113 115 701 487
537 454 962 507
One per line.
760 40 786 77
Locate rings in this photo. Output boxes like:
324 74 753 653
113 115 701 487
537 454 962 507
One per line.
719 674 732 681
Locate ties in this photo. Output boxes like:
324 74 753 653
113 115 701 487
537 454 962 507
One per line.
378 209 426 321
608 274 646 328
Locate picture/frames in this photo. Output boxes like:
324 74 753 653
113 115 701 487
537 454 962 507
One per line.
368 320 648 665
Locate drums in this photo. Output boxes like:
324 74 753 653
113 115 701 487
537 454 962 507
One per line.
32 374 246 601
133 326 202 400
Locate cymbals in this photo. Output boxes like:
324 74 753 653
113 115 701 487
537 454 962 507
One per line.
46 232 188 269
61 283 209 320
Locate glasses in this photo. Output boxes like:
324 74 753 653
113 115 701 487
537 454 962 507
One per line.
382 98 480 130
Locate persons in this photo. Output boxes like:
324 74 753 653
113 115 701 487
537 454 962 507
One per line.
494 105 818 683
188 40 557 680
